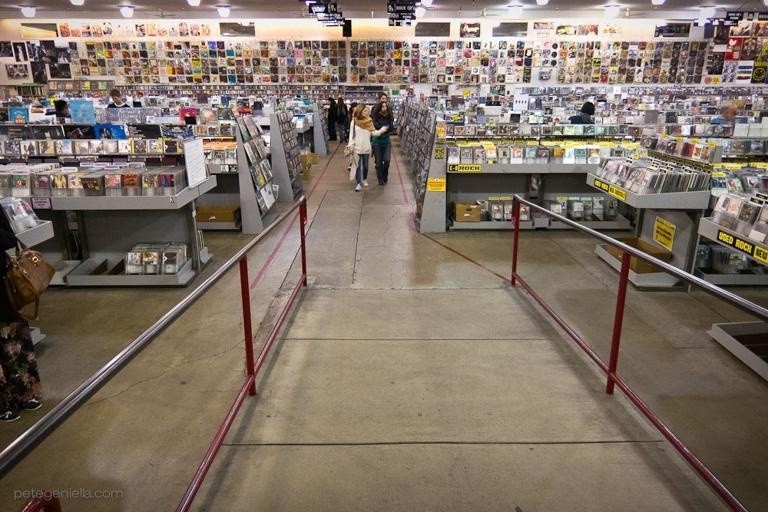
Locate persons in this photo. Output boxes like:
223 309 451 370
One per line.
53 100 70 117
322 92 396 191
567 102 595 124
104 90 131 108
710 103 737 125
0 202 44 422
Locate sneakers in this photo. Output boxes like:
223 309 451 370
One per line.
0 411 21 423
362 180 369 187
378 179 387 185
354 183 362 191
22 399 42 411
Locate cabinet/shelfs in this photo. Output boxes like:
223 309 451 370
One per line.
0 83 387 288
415 142 768 382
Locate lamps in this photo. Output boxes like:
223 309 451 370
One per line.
303 0 346 28
386 0 422 27
215 5 233 18
20 5 37 18
116 5 136 18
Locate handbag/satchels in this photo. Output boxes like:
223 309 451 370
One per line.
1 204 56 322
344 119 356 139
